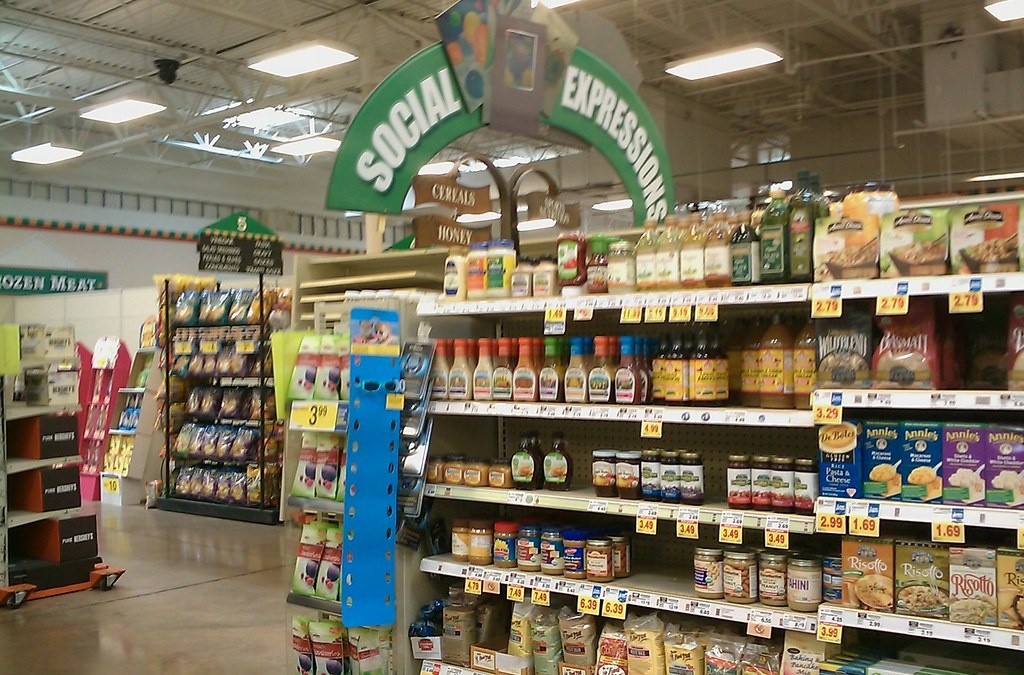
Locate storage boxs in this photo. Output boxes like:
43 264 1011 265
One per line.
471 633 593 675
6 324 99 562
813 202 1024 631
99 346 165 481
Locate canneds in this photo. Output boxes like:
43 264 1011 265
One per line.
427 456 515 488
443 229 635 302
451 518 629 582
696 544 843 608
594 448 820 517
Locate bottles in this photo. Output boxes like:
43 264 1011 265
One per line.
430 310 817 411
443 168 899 302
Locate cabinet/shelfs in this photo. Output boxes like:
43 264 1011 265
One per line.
286 301 393 675
156 272 280 526
812 271 1023 652
417 272 818 675
0 324 102 592
79 338 131 502
